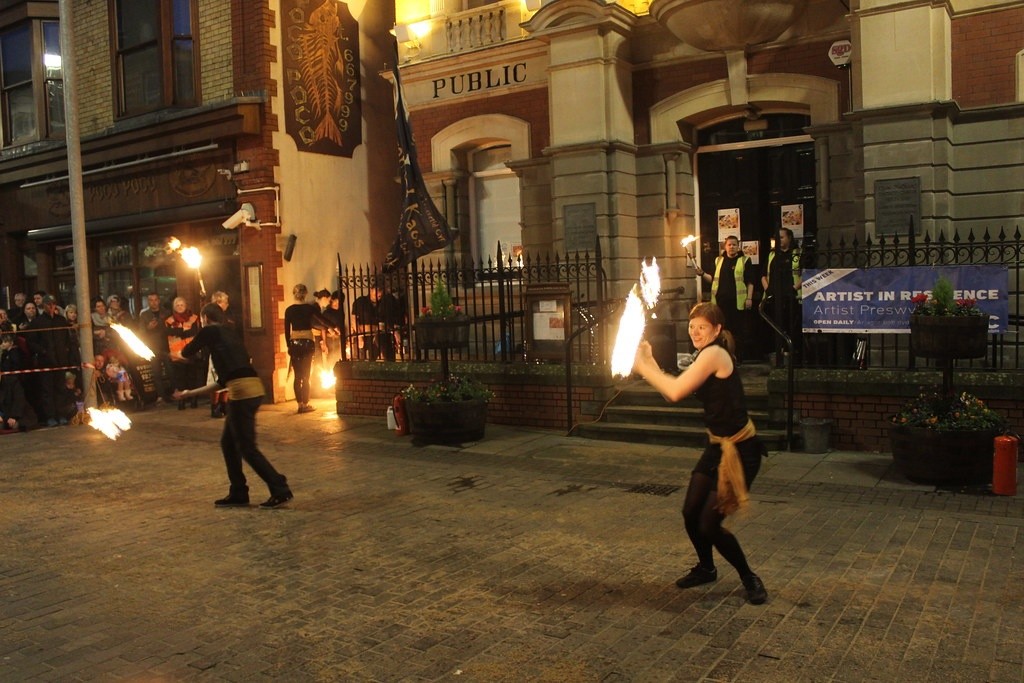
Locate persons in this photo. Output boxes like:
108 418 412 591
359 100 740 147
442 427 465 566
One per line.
0 289 199 431
310 289 345 398
352 286 408 362
173 302 294 508
632 303 768 605
284 284 335 414
206 291 238 417
759 227 802 331
696 235 755 367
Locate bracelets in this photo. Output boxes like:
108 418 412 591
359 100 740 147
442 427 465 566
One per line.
746 298 752 300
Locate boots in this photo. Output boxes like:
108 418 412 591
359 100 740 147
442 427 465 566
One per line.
220 403 229 413
258 474 294 508
125 389 133 400
117 390 126 401
211 405 222 417
214 485 250 506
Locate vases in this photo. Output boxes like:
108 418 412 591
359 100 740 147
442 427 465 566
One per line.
888 416 1006 487
404 400 489 446
414 318 470 349
799 417 833 455
910 313 989 360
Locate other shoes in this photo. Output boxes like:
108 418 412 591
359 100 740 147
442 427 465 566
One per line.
154 399 165 408
46 416 57 428
178 402 185 410
59 416 68 425
299 405 316 412
192 399 198 408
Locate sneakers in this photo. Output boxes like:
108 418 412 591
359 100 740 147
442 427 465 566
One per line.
676 563 718 588
742 573 768 603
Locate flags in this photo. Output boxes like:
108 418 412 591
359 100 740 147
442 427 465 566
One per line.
381 66 455 273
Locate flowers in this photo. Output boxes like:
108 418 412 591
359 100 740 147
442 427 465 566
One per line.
891 385 1009 430
912 270 988 317
419 277 468 321
400 373 496 406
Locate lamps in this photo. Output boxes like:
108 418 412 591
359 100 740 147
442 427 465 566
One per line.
742 102 763 122
525 0 542 13
394 24 419 43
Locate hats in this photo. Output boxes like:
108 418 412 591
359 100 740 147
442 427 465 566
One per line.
42 293 57 304
106 295 121 308
